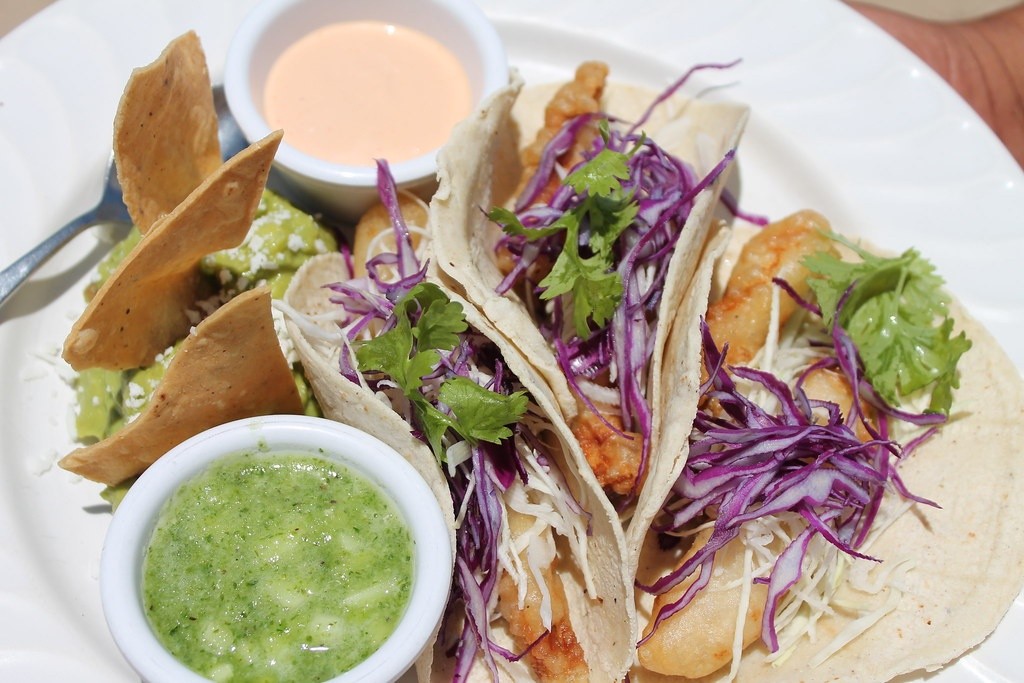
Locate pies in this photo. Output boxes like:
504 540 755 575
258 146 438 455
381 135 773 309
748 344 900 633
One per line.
626 215 1024 683
428 64 748 545
279 243 641 683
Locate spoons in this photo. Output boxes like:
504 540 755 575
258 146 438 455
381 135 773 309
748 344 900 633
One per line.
0 83 247 306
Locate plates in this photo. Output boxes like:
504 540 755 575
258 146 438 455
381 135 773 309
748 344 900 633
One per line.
0 0 1024 683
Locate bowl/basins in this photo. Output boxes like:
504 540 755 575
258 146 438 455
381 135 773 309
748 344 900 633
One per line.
224 1 507 222
100 414 451 683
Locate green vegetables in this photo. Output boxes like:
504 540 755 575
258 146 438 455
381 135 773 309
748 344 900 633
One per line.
473 113 647 342
347 281 529 467
802 232 974 419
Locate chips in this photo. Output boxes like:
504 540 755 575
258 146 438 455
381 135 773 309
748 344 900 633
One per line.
59 29 305 491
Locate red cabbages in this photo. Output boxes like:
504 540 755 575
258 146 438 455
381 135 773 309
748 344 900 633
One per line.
326 52 948 683
